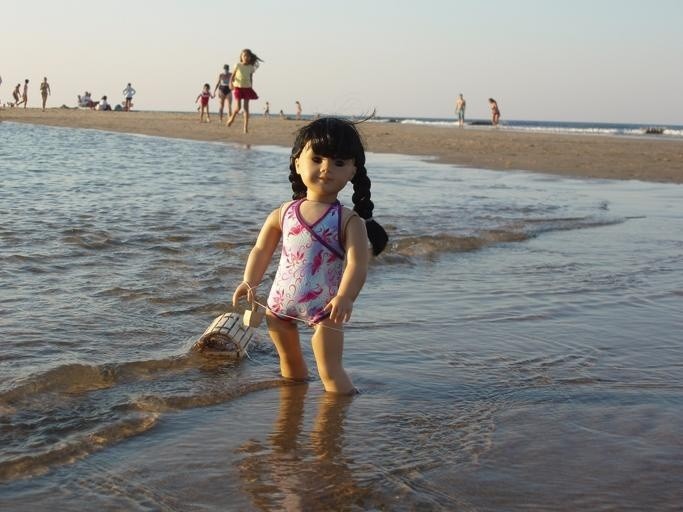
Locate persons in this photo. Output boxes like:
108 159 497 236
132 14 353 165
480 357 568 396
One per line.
264 102 270 119
488 98 500 128
80 91 111 111
40 76 51 112
213 64 232 126
294 100 302 120
17 79 29 109
280 110 291 119
455 93 466 129
123 83 136 110
195 83 214 123
225 48 263 134
12 84 22 105
234 115 389 393
